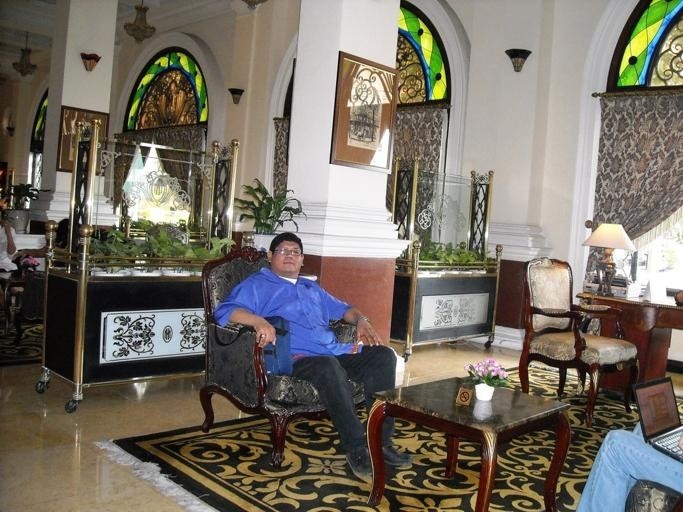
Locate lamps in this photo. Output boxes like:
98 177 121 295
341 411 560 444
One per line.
79 50 102 72
120 0 156 45
228 85 245 104
13 32 37 79
503 45 534 74
582 222 639 296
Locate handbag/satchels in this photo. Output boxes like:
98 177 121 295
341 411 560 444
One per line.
263 316 293 376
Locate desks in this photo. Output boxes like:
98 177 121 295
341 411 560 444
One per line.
574 290 682 395
0 269 45 326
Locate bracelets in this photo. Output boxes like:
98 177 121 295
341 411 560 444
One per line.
357 316 369 321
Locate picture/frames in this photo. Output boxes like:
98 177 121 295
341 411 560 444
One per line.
54 104 111 176
328 49 402 177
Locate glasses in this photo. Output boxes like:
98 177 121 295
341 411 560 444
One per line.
271 247 303 256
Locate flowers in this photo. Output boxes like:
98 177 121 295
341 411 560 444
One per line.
20 256 38 266
464 358 509 388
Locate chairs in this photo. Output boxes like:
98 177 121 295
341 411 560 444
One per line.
517 256 641 428
5 270 48 348
198 245 367 468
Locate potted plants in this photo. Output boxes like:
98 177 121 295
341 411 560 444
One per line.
232 178 308 250
0 182 51 234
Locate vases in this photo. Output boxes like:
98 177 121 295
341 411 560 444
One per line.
474 381 496 402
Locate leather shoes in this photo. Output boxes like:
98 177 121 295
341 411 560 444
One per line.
381 446 411 466
346 446 373 479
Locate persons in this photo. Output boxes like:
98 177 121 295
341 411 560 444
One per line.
576 417 683 512
211 231 411 484
0 206 21 292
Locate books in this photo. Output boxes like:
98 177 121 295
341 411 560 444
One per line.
583 268 652 301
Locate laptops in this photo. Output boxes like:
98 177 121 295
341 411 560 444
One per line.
631 377 683 464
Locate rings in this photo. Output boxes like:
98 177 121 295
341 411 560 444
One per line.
260 334 267 339
365 335 370 338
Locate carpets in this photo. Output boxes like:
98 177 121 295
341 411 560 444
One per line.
93 365 683 512
0 310 44 366
